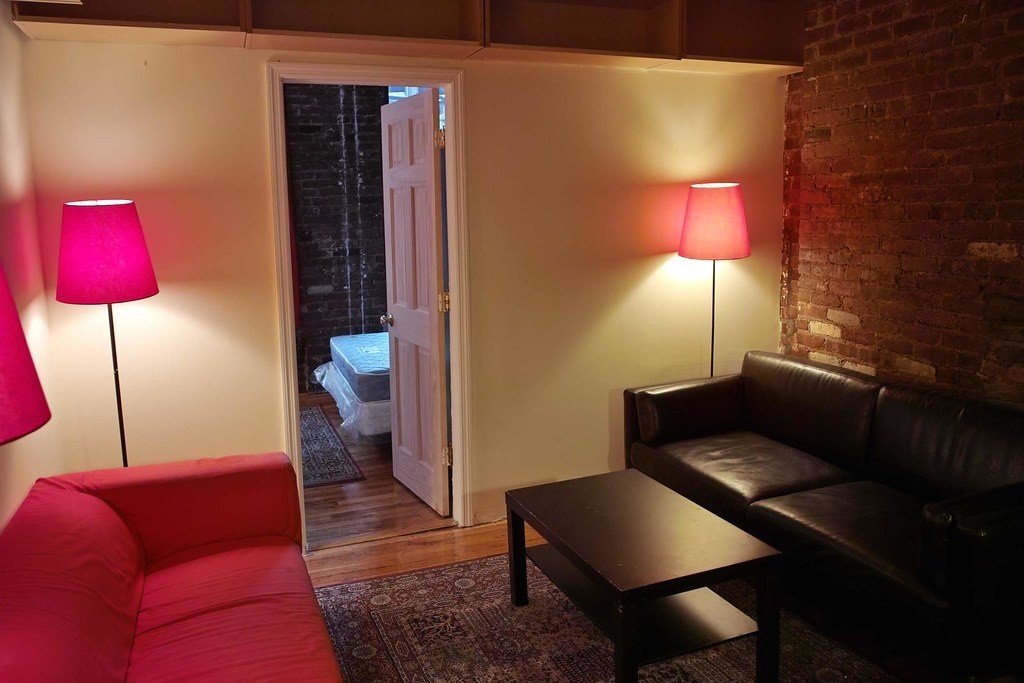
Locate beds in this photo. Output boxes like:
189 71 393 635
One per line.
309 331 391 446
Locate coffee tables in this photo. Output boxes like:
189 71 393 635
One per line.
505 468 784 683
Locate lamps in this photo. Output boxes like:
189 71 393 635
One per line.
677 183 752 377
54 197 162 468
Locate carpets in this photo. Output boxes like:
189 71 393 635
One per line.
300 404 367 489
314 551 904 683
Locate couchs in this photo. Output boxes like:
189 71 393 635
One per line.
623 350 1024 683
0 452 346 683
